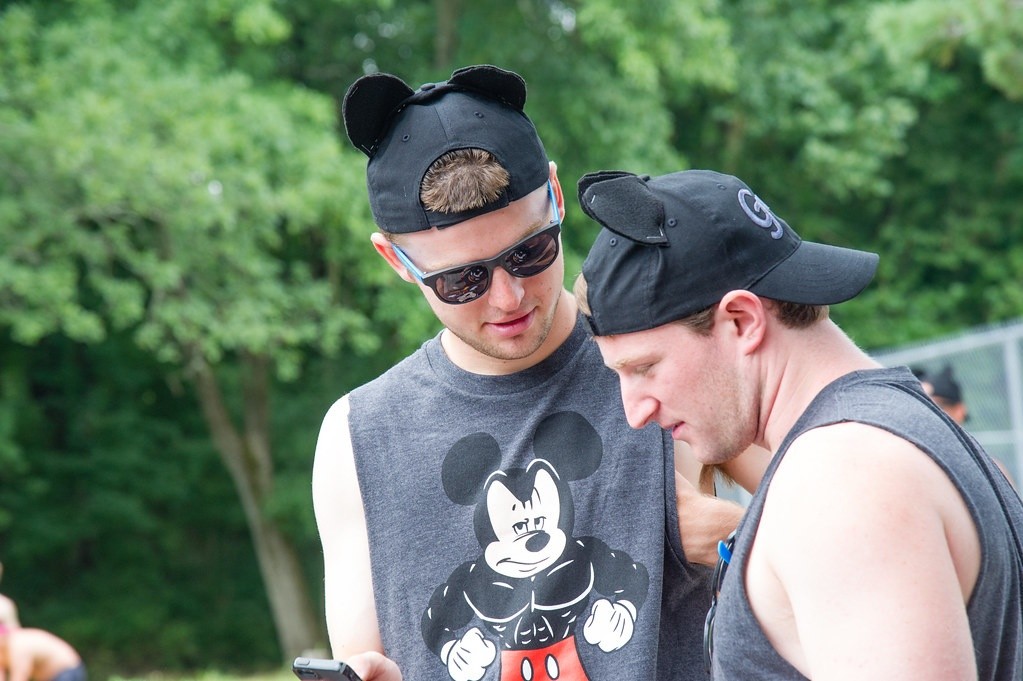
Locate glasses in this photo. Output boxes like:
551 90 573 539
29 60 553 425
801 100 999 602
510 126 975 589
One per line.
393 180 561 305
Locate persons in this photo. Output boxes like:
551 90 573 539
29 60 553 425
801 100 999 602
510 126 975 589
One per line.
0 563 21 632
311 64 772 680
0 627 88 681
910 364 1017 492
574 170 1023 680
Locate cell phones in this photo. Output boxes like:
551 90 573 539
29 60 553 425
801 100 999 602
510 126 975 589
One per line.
292 657 362 681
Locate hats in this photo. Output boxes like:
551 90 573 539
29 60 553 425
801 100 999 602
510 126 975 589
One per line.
343 64 549 234
577 170 880 336
907 364 972 422
